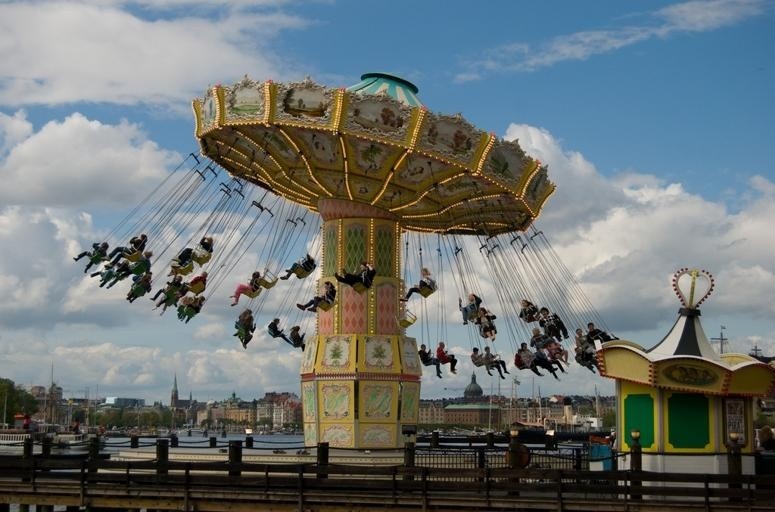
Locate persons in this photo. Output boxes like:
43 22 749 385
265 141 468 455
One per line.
760 425 775 450
